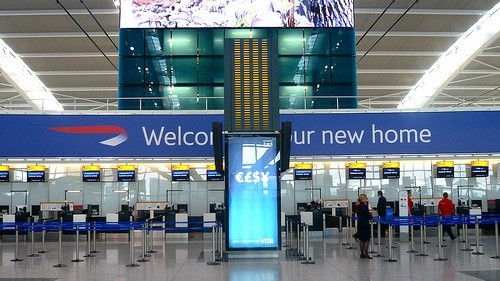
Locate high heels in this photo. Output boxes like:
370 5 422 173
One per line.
360 255 373 259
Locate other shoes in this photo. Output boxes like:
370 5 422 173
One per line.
451 237 456 240
353 234 357 241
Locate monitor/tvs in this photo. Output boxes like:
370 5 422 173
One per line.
207 170 224 181
31 205 40 216
437 167 454 178
0 205 9 215
177 204 187 213
27 171 45 182
383 168 400 179
295 169 312 180
154 210 165 217
467 200 483 208
83 171 100 182
349 168 366 179
118 171 135 182
138 210 150 218
91 205 99 215
172 170 189 181
471 166 489 177
0 171 9 182
297 203 307 213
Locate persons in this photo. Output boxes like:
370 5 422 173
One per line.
60 208 67 222
438 192 457 241
353 194 373 259
377 191 387 238
305 201 319 211
406 190 413 241
